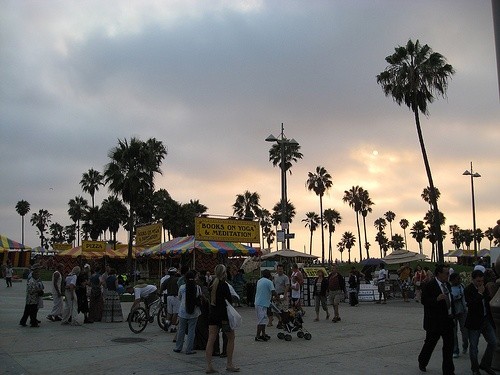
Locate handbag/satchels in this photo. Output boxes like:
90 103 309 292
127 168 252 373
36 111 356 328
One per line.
73 311 85 326
225 298 242 330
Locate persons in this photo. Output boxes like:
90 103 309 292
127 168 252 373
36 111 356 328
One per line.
130 283 158 323
417 261 500 375
349 263 434 311
18 267 45 328
160 262 247 374
254 264 306 344
1 259 14 288
312 265 349 323
48 263 130 326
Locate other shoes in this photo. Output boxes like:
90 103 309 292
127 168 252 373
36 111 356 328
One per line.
266 322 272 327
301 311 305 316
332 318 337 323
453 353 458 358
337 317 341 321
170 327 270 374
376 301 381 304
19 315 72 328
326 314 330 320
419 362 426 372
315 319 319 321
479 365 496 375
473 369 481 375
382 302 387 304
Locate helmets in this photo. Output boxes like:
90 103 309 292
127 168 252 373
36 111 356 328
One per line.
168 267 177 272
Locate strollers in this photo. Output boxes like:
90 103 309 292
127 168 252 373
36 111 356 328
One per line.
271 301 312 341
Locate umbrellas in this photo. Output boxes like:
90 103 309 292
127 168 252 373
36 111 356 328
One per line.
58 246 124 268
115 246 141 260
382 247 425 264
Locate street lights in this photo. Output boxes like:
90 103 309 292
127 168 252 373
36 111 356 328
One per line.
462 161 482 265
265 123 299 251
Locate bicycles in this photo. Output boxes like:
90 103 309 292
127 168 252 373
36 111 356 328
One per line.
127 293 170 334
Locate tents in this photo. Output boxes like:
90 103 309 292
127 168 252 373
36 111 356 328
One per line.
142 235 263 271
260 249 320 266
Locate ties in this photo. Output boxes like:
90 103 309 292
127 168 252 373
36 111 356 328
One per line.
441 283 450 310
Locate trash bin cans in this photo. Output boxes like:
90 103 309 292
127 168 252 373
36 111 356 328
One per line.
246 283 256 306
118 292 134 321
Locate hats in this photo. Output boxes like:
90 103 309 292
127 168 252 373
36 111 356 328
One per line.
84 263 90 267
